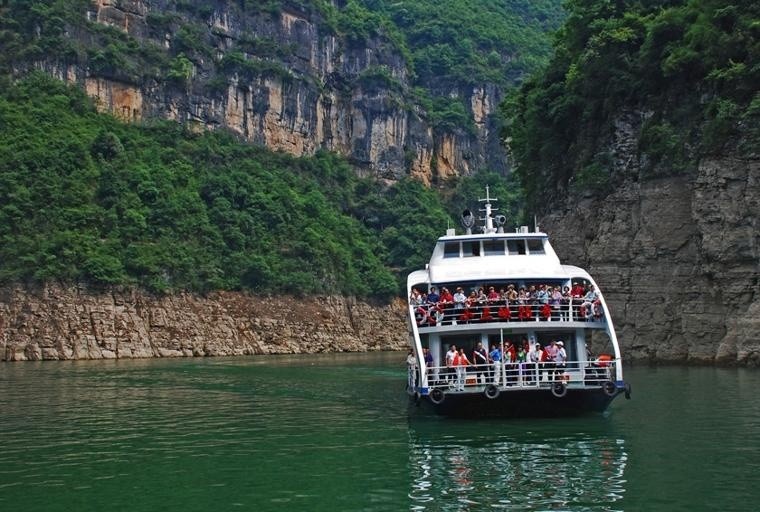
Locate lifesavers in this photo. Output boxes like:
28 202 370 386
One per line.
427 306 444 322
580 302 593 318
414 308 427 325
591 300 603 316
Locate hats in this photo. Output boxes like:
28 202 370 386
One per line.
456 282 579 291
504 341 565 346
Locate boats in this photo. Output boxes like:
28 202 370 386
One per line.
407 184 631 415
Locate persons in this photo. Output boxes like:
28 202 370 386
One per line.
585 343 594 373
407 348 433 367
446 341 567 391
594 354 615 378
410 280 597 304
